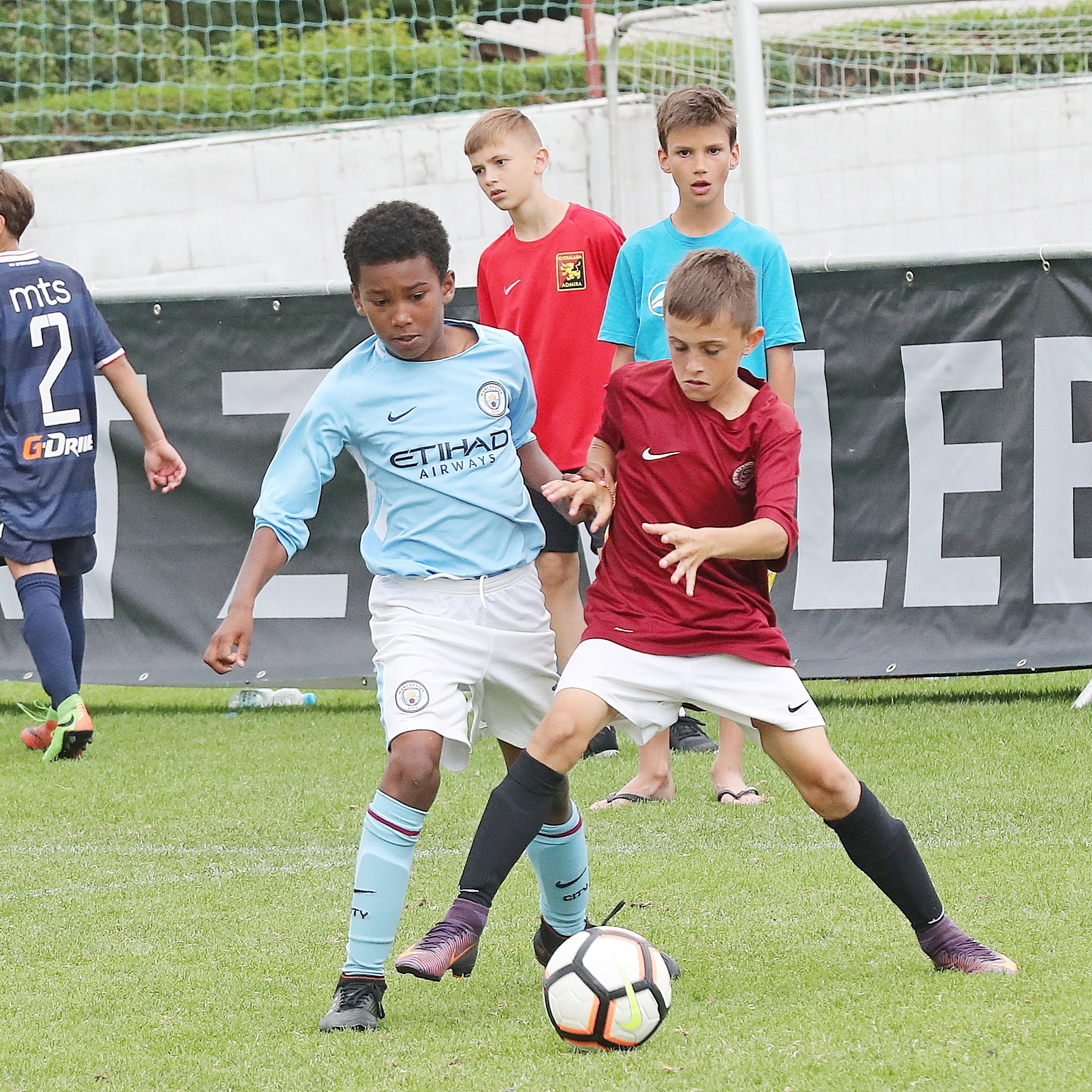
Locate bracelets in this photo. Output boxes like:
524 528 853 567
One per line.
606 483 616 512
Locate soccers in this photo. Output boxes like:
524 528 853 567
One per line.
542 926 672 1053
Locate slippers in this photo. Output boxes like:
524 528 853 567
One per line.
719 785 766 806
590 792 655 808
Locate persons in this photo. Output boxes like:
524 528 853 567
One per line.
582 84 806 811
392 248 1017 981
201 201 682 1031
0 164 186 760
463 108 718 761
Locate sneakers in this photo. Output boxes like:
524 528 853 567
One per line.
668 714 719 753
393 899 491 984
317 976 388 1033
915 915 1017 975
20 708 86 756
580 724 622 757
39 694 95 762
532 901 682 984
